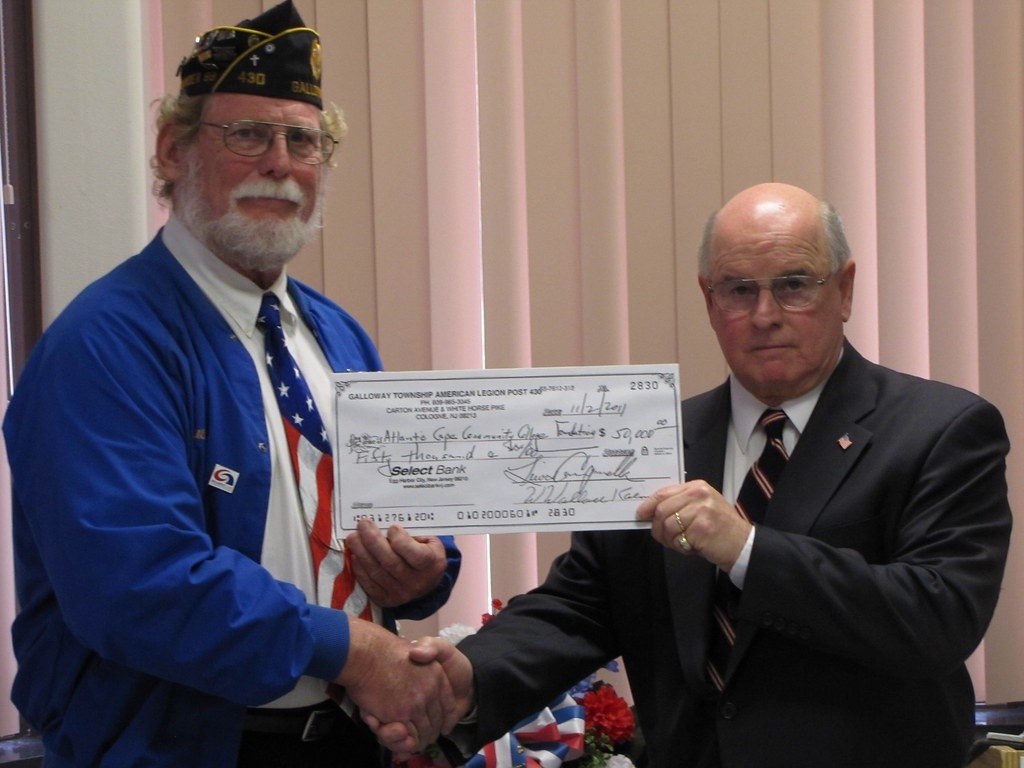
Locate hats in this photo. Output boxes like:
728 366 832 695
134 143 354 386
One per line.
176 1 322 110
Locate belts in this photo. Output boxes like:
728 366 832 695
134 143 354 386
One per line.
246 703 369 741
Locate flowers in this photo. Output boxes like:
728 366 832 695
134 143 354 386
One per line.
579 680 639 768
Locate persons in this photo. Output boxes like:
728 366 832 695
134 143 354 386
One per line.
358 180 1011 768
0 0 461 768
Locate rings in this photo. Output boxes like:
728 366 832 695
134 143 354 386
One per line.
678 534 692 551
675 512 685 532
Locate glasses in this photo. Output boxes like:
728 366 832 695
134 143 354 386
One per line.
703 265 841 321
190 118 342 167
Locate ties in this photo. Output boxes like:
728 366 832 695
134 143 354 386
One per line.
703 406 790 719
256 294 381 722
